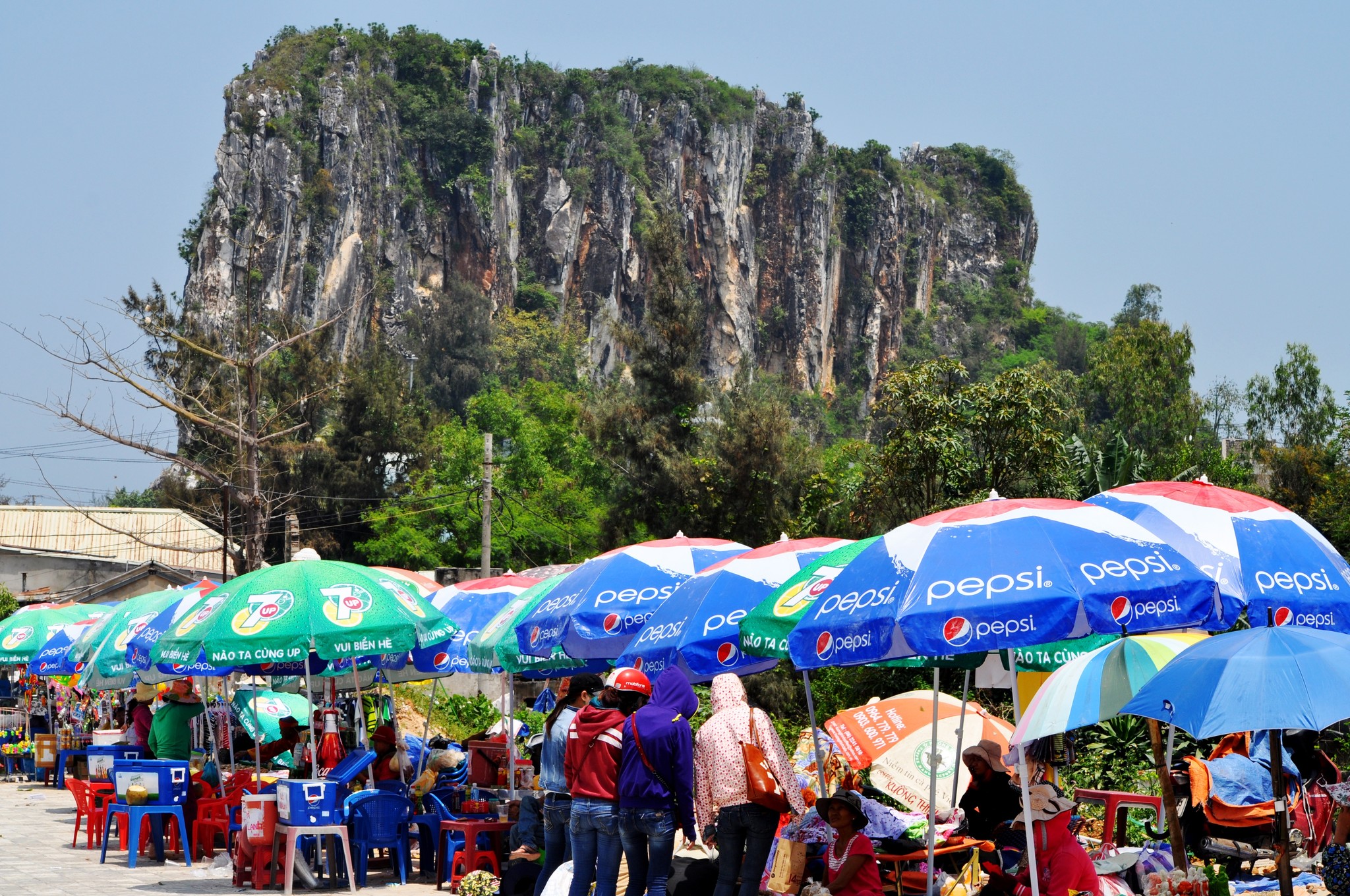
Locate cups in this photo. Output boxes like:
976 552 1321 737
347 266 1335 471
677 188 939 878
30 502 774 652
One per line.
201 856 214 868
497 804 509 823
164 859 180 871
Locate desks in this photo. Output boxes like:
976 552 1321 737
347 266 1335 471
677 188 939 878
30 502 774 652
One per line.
436 820 518 891
99 803 191 868
460 785 543 800
269 823 356 895
353 814 499 876
53 750 87 790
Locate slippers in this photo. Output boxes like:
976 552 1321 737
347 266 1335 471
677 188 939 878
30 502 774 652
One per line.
509 845 541 860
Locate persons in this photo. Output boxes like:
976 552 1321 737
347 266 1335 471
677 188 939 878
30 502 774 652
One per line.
2 668 1099 896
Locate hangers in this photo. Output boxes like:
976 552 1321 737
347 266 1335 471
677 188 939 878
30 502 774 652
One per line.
314 685 378 701
200 700 232 717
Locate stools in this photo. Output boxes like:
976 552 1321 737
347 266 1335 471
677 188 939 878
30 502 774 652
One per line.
232 831 286 890
449 851 501 894
45 767 65 786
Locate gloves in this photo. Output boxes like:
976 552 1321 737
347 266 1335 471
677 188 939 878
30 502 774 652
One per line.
988 871 1018 894
968 765 981 781
993 837 1008 851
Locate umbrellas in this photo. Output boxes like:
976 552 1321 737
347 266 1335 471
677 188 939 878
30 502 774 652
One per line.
0 474 1350 877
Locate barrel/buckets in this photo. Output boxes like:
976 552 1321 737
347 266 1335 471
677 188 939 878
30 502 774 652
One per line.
241 794 287 846
467 739 507 788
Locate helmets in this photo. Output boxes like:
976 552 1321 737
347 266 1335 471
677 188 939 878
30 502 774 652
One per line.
605 667 651 697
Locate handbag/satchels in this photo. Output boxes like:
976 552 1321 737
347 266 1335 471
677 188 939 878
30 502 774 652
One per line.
389 751 400 772
201 741 219 788
672 791 683 829
396 731 410 751
738 708 791 814
426 748 465 771
401 751 414 778
767 816 806 894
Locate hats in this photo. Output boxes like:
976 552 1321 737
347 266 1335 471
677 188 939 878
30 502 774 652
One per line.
1010 784 1078 831
962 739 1011 773
815 789 869 831
369 726 397 745
569 672 605 697
279 716 298 729
160 680 202 703
133 682 162 702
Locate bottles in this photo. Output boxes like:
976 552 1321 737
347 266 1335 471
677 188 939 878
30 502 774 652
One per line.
316 709 349 789
354 781 362 792
54 719 93 751
293 731 312 767
189 750 204 772
409 785 417 815
452 782 461 813
465 784 471 801
41 695 45 705
470 783 479 802
415 785 424 814
497 757 535 790
488 799 501 813
364 780 372 790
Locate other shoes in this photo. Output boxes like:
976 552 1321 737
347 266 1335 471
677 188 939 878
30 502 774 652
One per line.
147 843 180 860
183 845 218 860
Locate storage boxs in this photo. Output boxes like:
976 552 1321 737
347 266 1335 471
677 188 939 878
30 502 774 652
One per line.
86 745 144 783
34 733 74 767
92 729 127 745
113 759 189 805
276 749 377 826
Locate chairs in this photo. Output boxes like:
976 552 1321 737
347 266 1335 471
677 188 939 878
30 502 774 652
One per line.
297 780 415 887
65 770 276 863
422 759 493 882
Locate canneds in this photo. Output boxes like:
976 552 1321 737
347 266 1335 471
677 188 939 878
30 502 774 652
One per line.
488 799 500 814
60 729 71 749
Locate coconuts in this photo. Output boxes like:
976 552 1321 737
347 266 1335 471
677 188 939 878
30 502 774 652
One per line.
126 785 148 805
